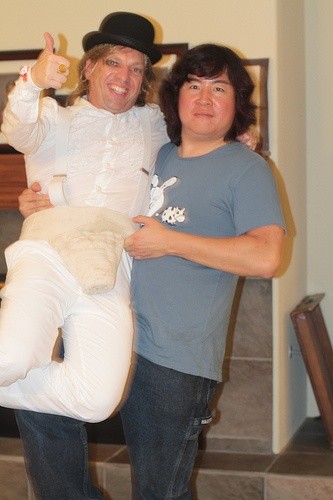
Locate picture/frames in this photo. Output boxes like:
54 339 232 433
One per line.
0 43 269 158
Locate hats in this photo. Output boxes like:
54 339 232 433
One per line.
82 11 162 66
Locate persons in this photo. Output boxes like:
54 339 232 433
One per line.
0 11 257 422
11 43 286 499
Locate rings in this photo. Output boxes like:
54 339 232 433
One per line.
58 63 66 74
